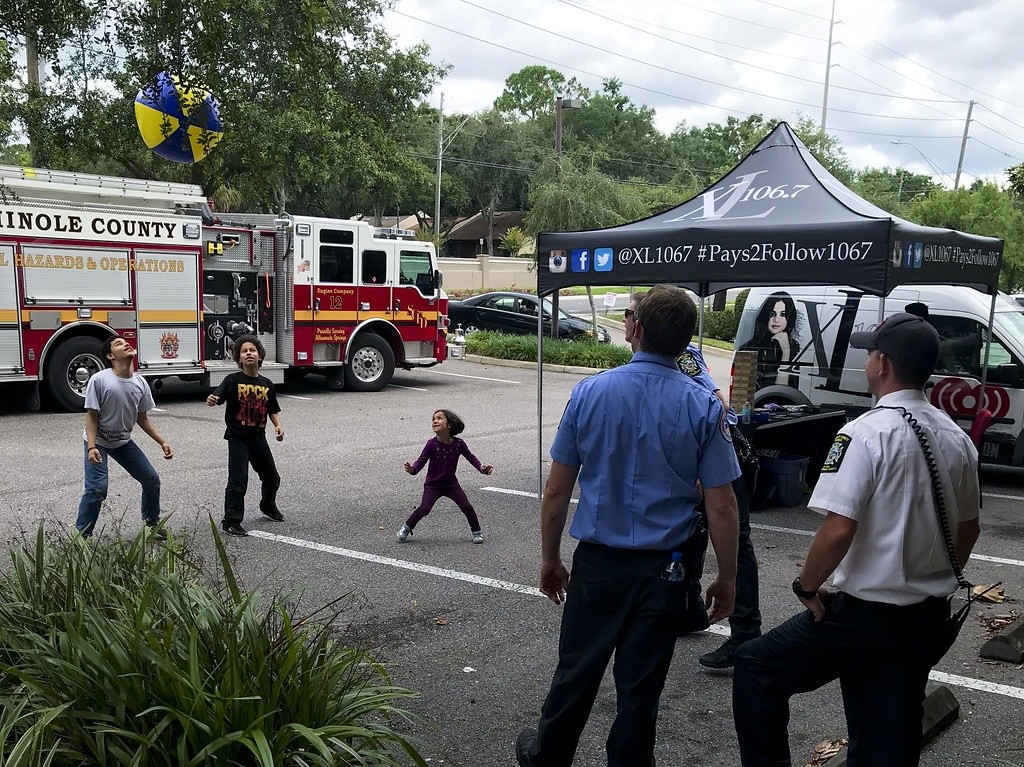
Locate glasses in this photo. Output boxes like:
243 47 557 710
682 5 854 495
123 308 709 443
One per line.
632 311 646 330
625 309 634 319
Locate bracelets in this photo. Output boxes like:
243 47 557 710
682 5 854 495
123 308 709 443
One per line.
87 447 96 453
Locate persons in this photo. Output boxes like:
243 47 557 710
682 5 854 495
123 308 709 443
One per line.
75 335 177 542
515 285 742 767
205 334 285 537
730 310 981 767
395 410 494 544
623 292 762 668
740 291 801 390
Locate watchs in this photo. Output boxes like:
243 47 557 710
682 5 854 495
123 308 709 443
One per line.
792 577 817 598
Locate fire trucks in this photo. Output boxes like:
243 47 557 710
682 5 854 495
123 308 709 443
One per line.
1 166 451 414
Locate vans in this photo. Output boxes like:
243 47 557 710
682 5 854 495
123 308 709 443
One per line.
728 282 1024 489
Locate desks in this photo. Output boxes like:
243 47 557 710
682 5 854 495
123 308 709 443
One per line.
745 409 847 431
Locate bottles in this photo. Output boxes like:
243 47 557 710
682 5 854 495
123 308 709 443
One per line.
742 402 751 424
660 552 685 582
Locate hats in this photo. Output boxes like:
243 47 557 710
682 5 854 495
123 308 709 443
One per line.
850 313 940 376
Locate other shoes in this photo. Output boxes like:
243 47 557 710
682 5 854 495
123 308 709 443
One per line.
258 501 285 522
144 516 168 539
221 520 249 537
677 598 711 636
472 531 484 543
396 524 413 541
699 625 762 669
516 729 538 767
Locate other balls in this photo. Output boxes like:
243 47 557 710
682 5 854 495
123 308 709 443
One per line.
134 72 230 165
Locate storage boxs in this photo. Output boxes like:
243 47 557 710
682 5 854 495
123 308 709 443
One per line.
729 351 759 411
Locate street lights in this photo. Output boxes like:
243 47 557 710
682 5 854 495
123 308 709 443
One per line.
550 95 582 341
434 89 492 257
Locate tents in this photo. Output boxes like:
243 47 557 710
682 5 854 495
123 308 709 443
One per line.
536 119 1005 503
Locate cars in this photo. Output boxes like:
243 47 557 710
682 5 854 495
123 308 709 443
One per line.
448 292 612 346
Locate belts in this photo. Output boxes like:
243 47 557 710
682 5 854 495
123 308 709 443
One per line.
581 542 666 560
